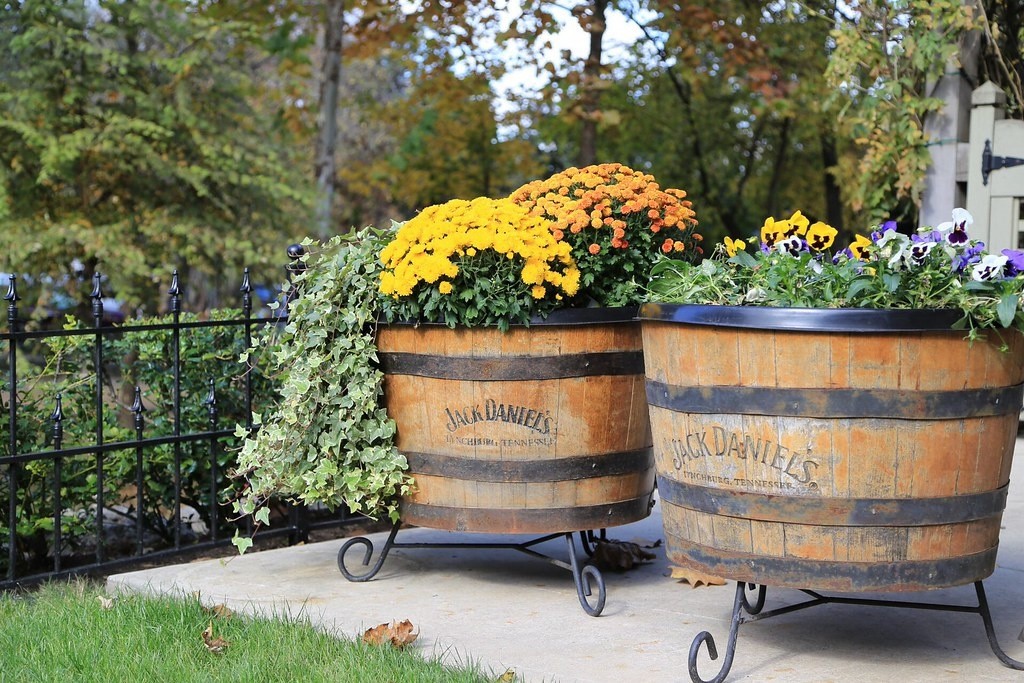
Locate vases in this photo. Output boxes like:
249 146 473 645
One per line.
338 305 656 618
632 304 1024 683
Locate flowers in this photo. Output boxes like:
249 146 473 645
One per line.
625 207 1024 355
219 163 704 565
